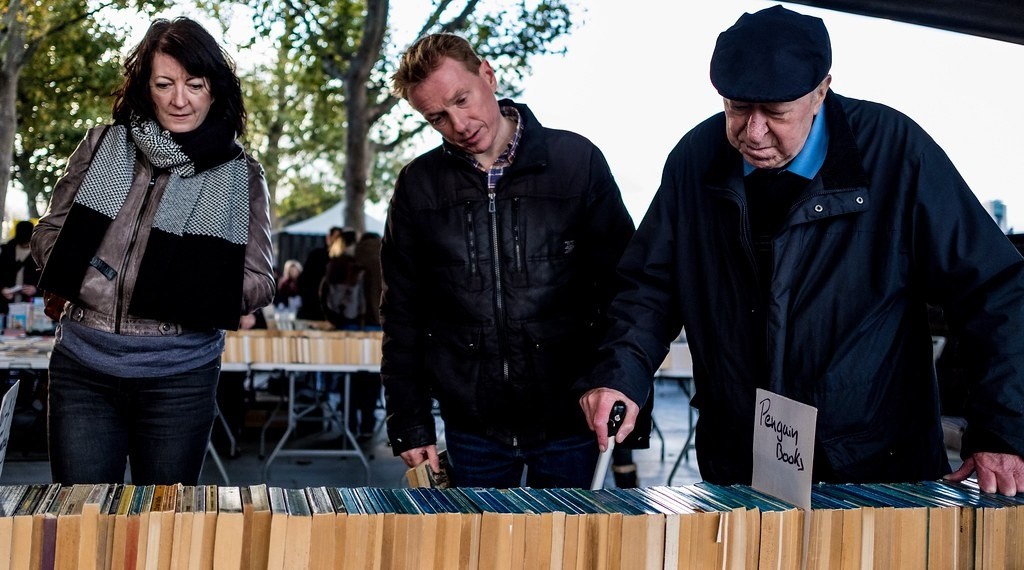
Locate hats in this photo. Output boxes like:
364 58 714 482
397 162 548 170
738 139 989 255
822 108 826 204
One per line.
710 4 833 101
16 221 34 242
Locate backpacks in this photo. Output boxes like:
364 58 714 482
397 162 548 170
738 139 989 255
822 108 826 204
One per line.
326 260 370 329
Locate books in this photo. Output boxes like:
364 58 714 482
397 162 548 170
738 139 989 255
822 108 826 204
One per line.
218 317 384 367
406 449 451 487
0 285 55 367
0 479 1023 570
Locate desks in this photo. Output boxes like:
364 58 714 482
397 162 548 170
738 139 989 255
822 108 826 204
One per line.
208 362 249 485
0 337 56 369
288 400 298 404
249 363 382 487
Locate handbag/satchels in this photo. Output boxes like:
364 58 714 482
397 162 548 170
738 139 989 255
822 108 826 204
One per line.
43 124 113 321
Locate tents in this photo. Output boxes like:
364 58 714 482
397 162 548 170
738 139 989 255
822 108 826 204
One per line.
279 200 388 269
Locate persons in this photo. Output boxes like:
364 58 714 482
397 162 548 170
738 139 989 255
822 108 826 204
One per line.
0 219 44 317
271 225 386 440
27 20 278 489
377 35 655 487
207 307 266 452
576 3 1023 500
